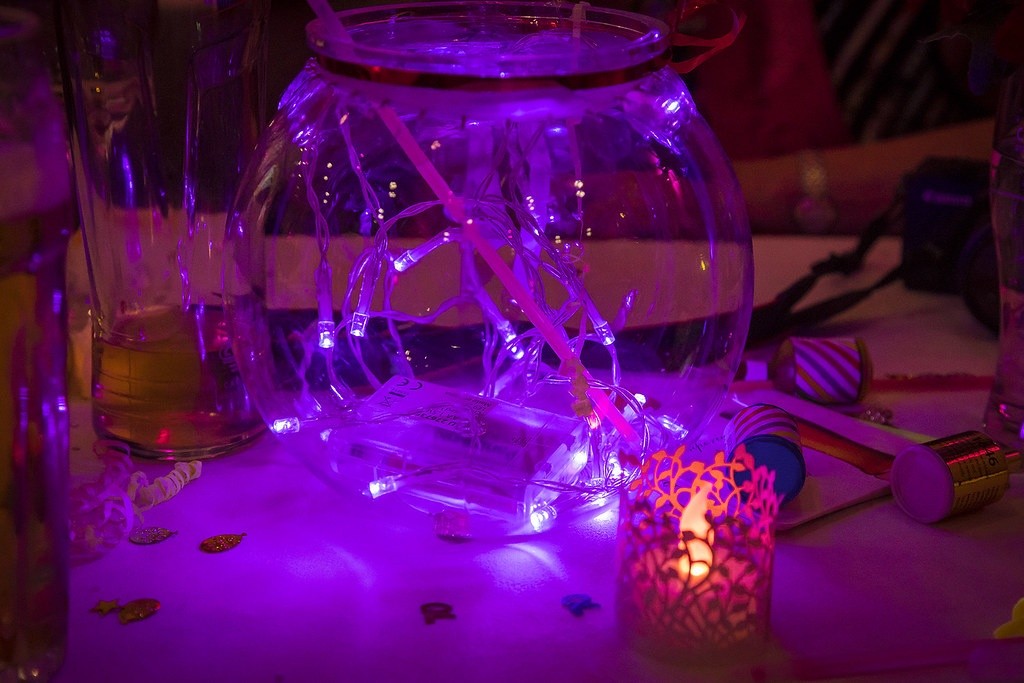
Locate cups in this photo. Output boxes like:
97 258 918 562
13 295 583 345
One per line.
980 130 1024 451
53 0 265 464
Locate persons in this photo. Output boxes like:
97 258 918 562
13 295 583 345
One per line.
282 0 1018 238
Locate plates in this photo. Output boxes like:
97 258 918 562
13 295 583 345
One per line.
231 237 903 371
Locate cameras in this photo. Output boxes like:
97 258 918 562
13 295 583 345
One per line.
898 154 1000 335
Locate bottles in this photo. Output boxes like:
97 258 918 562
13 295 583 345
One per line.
0 8 70 683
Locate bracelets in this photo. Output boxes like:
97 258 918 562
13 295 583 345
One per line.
791 141 837 237
